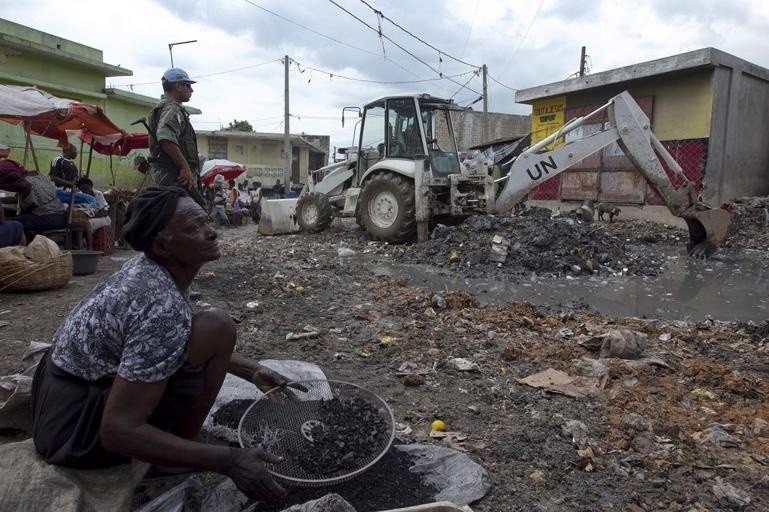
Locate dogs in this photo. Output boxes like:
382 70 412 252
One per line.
594 203 621 223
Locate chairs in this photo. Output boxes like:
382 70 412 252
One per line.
35 185 79 252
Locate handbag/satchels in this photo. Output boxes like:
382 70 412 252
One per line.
69 206 95 222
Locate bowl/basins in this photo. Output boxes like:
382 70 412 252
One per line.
72 249 104 275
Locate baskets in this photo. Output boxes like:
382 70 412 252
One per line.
0 249 73 289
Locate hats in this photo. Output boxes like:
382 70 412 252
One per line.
162 68 197 84
133 156 148 169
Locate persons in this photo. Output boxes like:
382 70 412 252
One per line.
206 174 299 229
148 67 200 294
0 202 27 247
33 184 307 505
201 158 247 186
406 114 436 150
76 177 111 251
1 159 66 232
0 142 11 161
49 142 78 181
132 156 149 173
